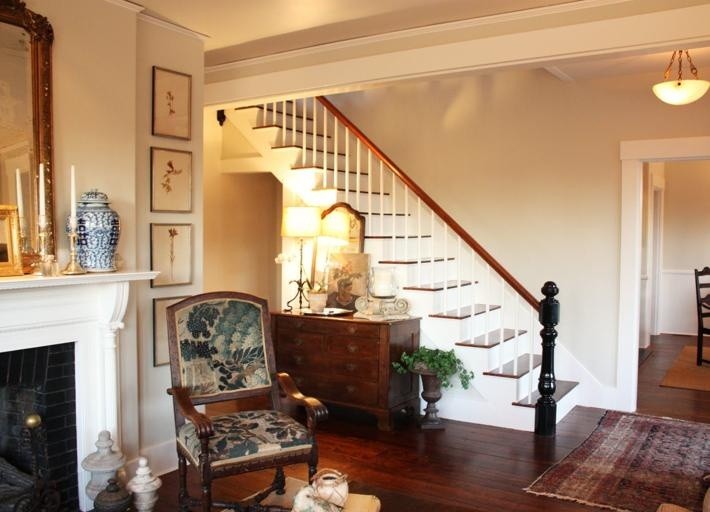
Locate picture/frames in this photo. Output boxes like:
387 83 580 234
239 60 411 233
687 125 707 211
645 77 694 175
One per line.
149 66 195 369
1 206 24 277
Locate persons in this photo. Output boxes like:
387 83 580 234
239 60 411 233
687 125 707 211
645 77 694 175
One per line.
327 279 360 311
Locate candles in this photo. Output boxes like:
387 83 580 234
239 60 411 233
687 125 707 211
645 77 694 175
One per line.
16 168 24 217
70 165 77 216
39 163 45 215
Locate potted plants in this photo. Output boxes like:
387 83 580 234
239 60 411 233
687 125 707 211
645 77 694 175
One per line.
391 346 474 430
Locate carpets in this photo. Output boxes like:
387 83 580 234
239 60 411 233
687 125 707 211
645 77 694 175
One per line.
659 345 710 392
521 409 710 512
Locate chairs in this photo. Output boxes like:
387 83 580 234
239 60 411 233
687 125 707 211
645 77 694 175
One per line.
165 291 330 511
694 267 710 368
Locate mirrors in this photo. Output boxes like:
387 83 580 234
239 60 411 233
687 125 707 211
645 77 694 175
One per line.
310 202 367 308
1 1 56 275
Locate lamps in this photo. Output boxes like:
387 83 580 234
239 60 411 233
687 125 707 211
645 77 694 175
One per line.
652 50 710 107
281 206 322 312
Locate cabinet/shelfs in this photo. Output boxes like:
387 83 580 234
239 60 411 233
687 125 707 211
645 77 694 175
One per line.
270 309 423 432
